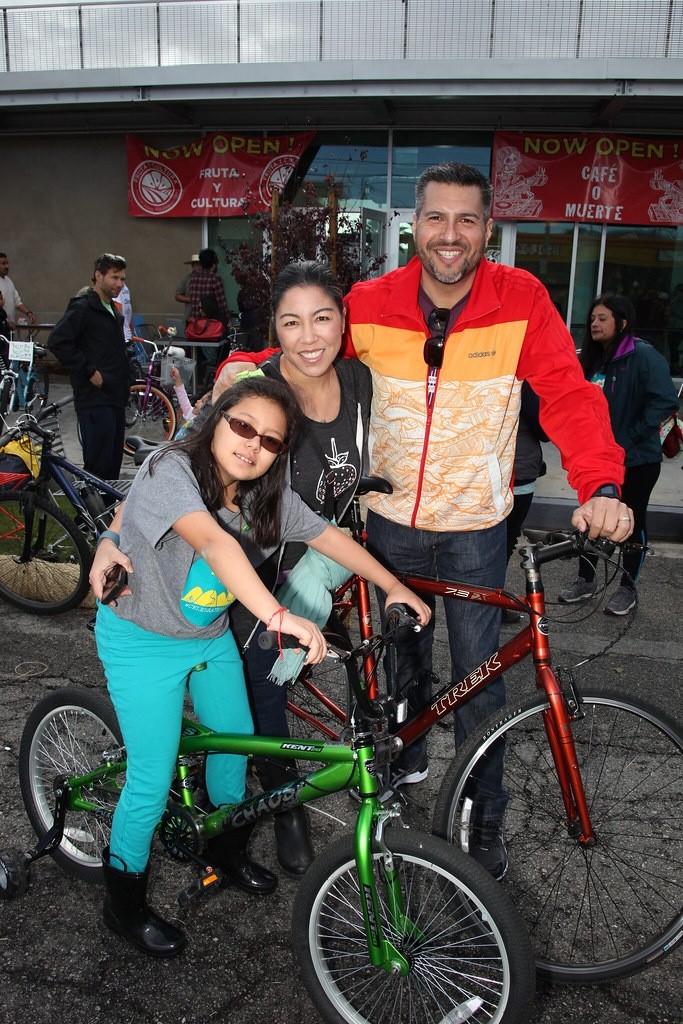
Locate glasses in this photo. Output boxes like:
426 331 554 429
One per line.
97 252 126 270
219 410 288 454
424 307 450 370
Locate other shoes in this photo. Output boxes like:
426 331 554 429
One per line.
503 608 520 623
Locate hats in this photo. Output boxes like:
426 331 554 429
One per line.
183 254 200 264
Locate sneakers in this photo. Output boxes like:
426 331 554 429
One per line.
349 742 430 803
471 822 509 881
558 576 597 602
603 586 636 615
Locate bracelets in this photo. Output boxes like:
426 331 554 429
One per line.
267 605 291 631
27 310 33 316
97 530 120 548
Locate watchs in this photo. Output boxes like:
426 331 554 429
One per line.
592 482 620 500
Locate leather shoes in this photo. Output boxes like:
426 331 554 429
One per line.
275 806 314 880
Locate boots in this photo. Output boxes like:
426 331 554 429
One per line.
207 804 279 895
102 846 186 957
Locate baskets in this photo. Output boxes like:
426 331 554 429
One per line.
8 342 33 361
160 355 196 391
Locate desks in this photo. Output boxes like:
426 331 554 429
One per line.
154 335 228 396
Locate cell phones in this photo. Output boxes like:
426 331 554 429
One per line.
101 565 127 604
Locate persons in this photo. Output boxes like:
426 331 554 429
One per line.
168 363 213 443
213 163 635 886
175 254 203 329
46 249 135 517
561 292 681 616
95 378 432 958
189 249 234 392
501 378 550 624
88 258 374 877
0 252 37 371
235 266 273 355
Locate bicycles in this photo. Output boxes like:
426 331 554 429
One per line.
268 475 683 986
1 602 533 1024
0 391 175 616
0 313 260 461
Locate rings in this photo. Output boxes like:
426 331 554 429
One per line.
619 517 630 521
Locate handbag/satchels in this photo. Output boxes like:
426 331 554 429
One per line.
186 316 224 340
